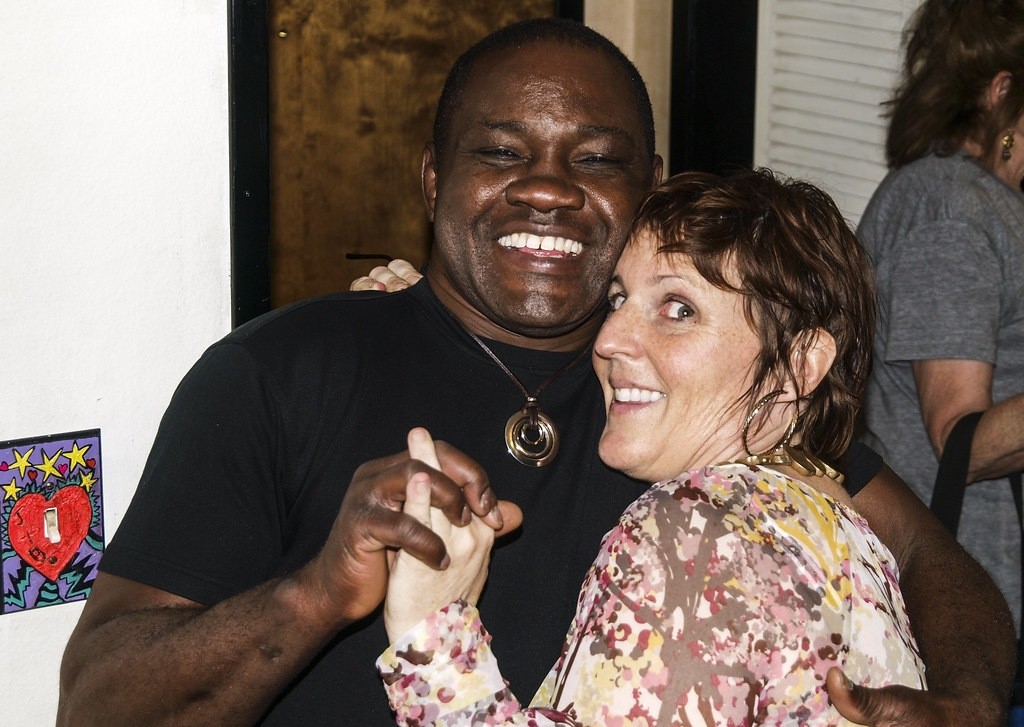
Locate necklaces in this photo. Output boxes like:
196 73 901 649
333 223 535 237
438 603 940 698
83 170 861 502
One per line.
426 278 596 468
711 445 844 485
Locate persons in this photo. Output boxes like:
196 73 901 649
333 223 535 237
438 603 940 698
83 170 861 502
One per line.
54 0 1024 727
